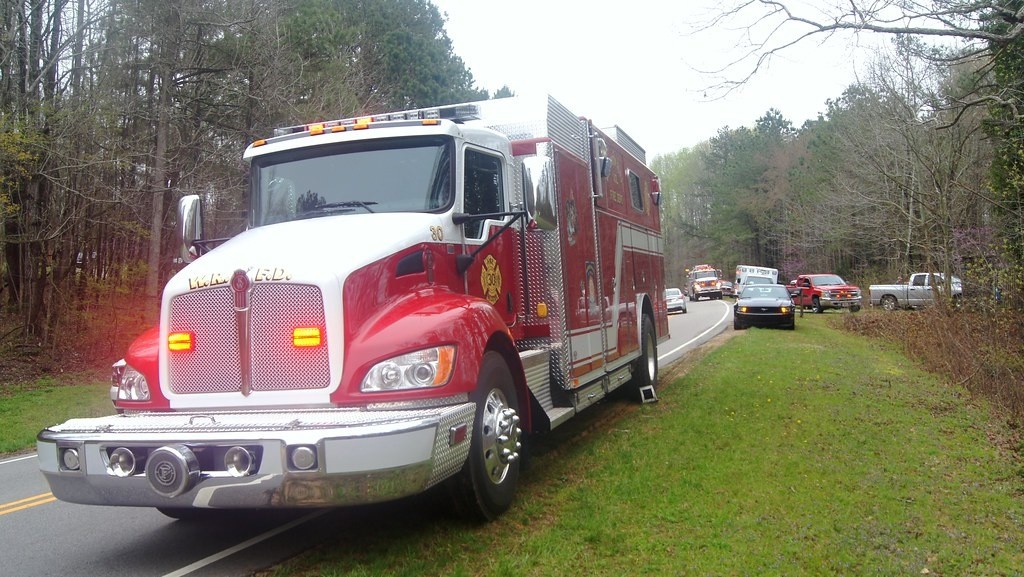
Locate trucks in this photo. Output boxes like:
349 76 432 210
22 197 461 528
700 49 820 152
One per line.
733 265 778 297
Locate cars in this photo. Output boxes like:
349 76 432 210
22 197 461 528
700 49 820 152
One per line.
663 288 687 314
721 281 734 298
734 284 795 331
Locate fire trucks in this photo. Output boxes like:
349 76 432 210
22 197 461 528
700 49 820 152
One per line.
37 93 670 521
685 265 723 302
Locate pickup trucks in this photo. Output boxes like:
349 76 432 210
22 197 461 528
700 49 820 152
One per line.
786 274 862 314
868 272 962 312
